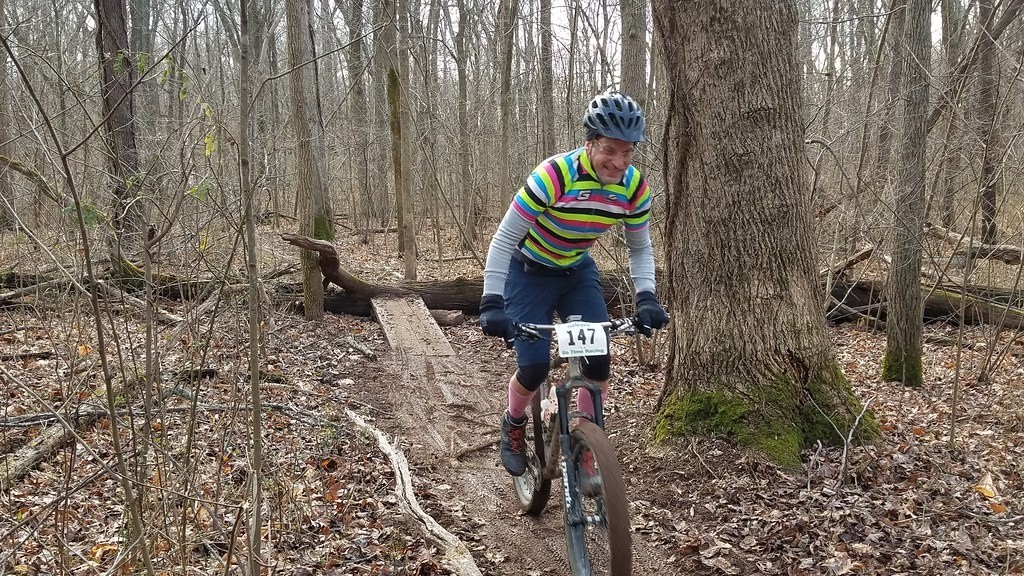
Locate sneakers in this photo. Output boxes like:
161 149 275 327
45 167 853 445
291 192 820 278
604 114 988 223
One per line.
499 408 529 477
570 435 602 497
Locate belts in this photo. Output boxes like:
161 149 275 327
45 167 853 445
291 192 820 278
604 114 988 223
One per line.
512 250 580 278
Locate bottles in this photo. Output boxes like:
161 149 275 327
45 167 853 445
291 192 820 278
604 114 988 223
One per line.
541 381 564 428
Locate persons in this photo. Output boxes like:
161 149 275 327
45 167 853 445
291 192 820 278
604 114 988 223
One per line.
477 92 668 494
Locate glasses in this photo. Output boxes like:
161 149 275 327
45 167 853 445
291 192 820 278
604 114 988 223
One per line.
590 137 635 157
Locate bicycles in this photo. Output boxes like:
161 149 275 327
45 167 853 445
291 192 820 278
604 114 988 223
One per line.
503 302 669 576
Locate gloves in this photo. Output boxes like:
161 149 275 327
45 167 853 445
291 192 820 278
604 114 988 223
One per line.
478 293 514 349
631 291 670 338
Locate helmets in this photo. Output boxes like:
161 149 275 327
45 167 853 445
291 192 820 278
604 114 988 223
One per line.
583 90 646 143
